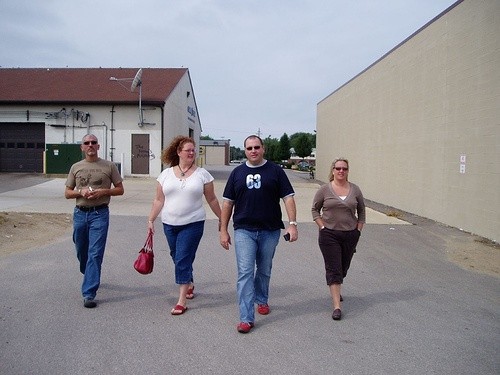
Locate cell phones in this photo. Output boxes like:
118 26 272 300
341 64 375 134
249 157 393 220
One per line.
284 233 290 241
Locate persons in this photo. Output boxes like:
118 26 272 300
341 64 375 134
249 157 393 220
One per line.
220 135 300 336
146 134 224 316
65 132 126 308
310 155 367 321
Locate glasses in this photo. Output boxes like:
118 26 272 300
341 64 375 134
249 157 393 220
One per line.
83 141 97 145
181 149 197 152
246 146 260 150
334 167 347 171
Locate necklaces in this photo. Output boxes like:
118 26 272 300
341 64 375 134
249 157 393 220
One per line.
177 163 194 176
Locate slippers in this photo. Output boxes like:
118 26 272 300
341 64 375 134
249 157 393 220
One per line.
185 286 194 299
172 305 188 315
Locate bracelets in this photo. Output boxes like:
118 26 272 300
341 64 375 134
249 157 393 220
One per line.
289 221 297 226
320 225 325 229
78 189 82 197
357 228 361 231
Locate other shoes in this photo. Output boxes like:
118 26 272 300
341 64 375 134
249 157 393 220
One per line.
83 298 96 307
331 308 342 319
339 294 343 302
258 304 270 315
237 322 253 333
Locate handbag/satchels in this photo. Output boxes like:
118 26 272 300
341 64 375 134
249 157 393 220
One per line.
133 229 154 275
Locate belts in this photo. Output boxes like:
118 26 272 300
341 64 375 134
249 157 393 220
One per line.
75 204 108 212
